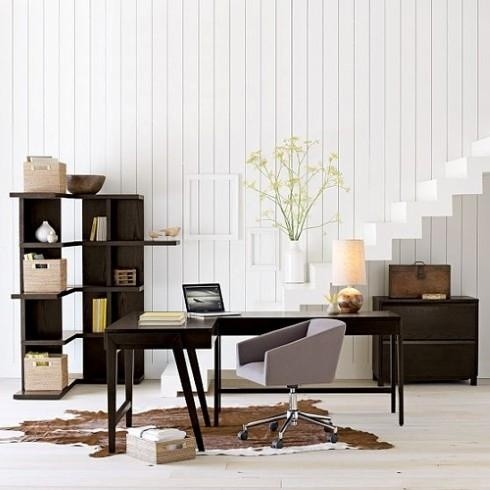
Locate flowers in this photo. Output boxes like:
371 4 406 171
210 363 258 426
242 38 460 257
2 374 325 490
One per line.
244 137 350 240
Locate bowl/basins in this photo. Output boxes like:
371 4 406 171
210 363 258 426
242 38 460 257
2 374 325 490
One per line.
66 174 106 194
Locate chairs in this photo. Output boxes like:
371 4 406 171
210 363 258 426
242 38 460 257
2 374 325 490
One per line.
236 317 346 449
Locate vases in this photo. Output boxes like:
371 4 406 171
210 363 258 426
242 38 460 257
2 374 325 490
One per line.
284 241 307 284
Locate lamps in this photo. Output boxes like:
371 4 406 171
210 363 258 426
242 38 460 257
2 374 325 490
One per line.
332 238 365 313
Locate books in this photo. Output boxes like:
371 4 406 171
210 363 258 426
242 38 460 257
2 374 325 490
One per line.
138 312 185 326
90 216 107 241
24 352 49 366
24 252 46 268
92 298 108 333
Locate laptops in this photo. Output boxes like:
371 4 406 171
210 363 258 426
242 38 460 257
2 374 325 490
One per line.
183 283 240 317
187 315 240 320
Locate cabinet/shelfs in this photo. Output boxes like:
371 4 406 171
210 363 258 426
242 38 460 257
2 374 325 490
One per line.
10 193 145 400
372 295 479 387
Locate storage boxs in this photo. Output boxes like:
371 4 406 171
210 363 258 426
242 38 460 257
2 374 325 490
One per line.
126 432 197 465
389 264 451 300
24 156 66 193
24 354 67 391
23 260 66 292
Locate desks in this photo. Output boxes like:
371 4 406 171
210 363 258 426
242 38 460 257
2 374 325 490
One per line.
104 312 404 454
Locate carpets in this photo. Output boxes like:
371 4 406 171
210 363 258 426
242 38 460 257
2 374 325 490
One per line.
0 399 393 458
